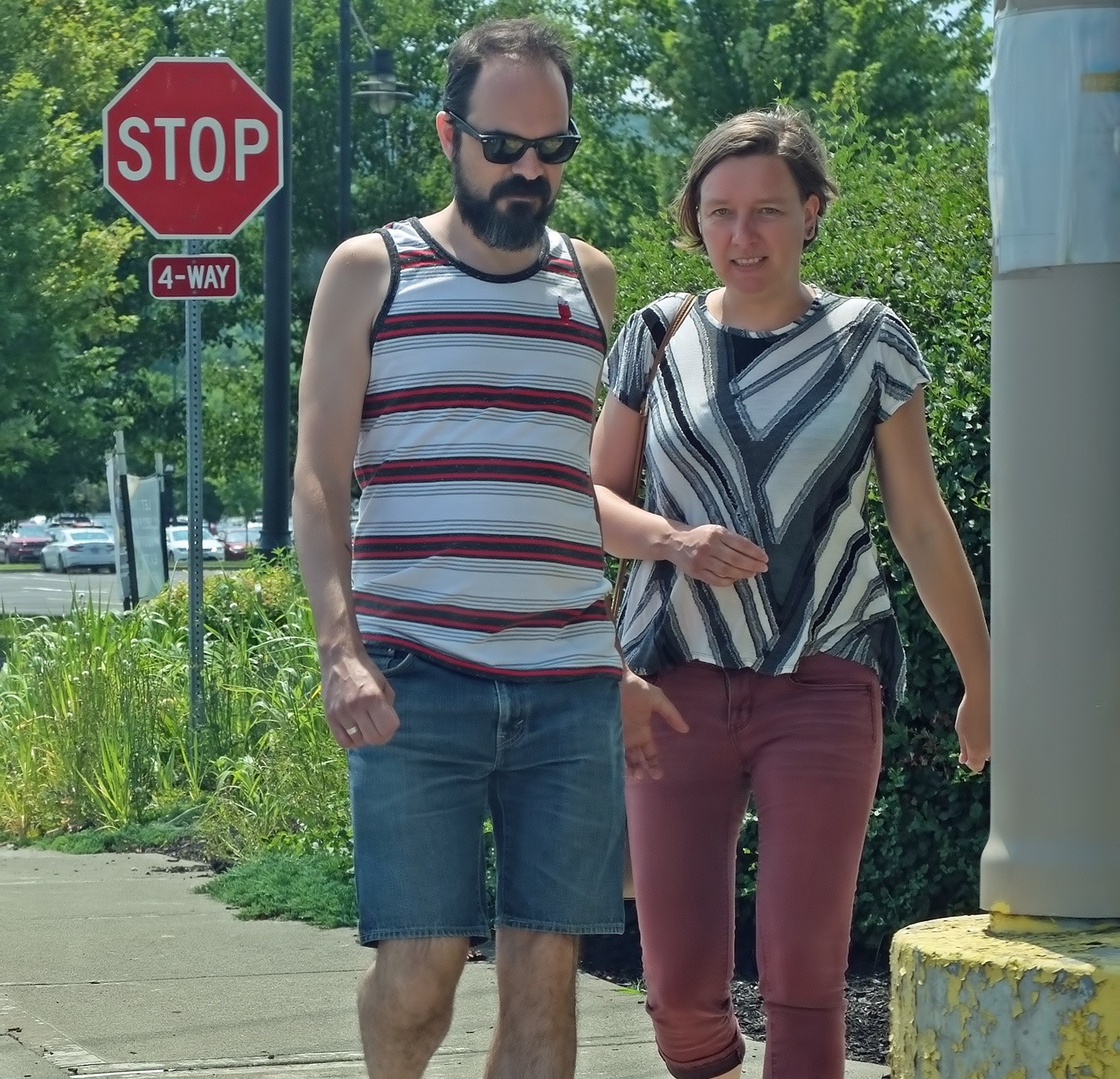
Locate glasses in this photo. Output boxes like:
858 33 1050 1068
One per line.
449 110 583 164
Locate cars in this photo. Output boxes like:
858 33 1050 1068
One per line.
38 527 116 574
165 511 295 564
0 511 116 563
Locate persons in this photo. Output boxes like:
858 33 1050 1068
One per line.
289 18 619 1079
592 114 992 1079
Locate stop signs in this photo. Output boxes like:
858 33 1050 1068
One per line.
101 55 285 241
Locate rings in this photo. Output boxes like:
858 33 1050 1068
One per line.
346 725 358 736
